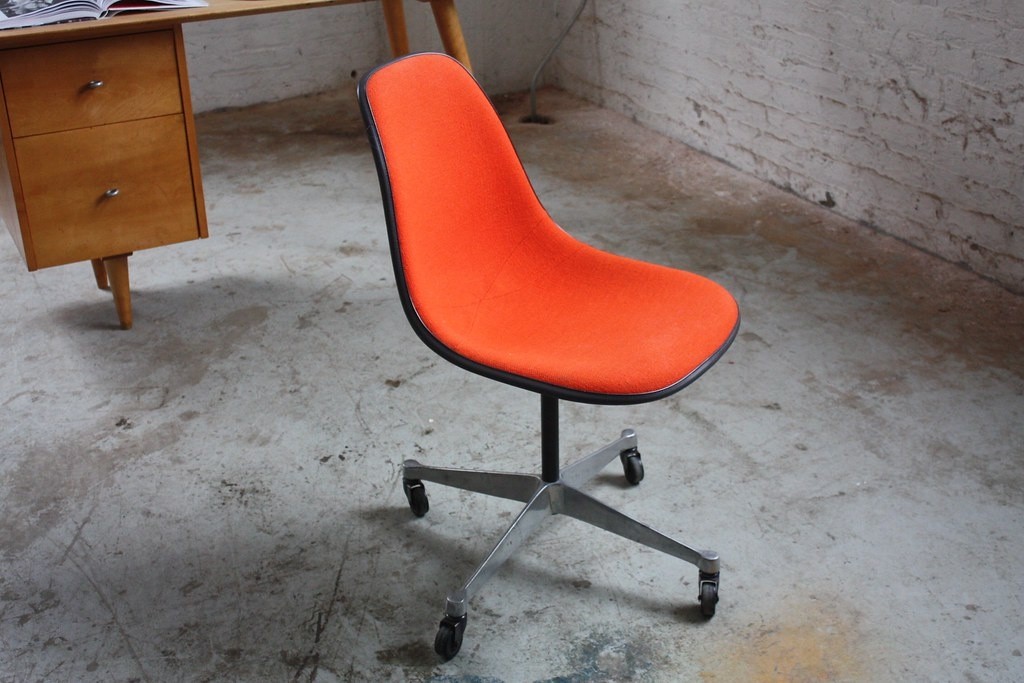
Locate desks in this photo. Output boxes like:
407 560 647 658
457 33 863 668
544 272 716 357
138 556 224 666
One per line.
0 0 479 332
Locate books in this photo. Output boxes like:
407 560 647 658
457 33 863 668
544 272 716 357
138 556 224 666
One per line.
0 0 208 30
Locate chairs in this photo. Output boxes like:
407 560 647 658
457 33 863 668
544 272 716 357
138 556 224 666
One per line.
356 52 741 662
